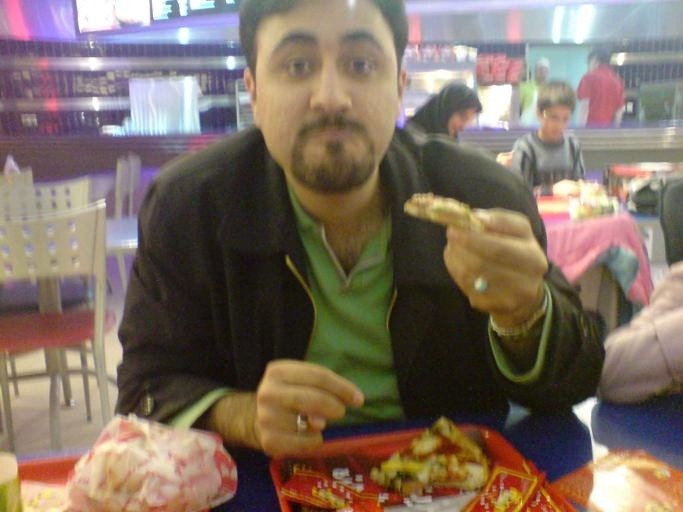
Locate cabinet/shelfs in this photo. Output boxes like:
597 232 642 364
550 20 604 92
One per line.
609 52 682 99
1 54 246 116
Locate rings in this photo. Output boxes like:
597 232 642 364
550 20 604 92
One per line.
474 273 490 293
295 413 313 434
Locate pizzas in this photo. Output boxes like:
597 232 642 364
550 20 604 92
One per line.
400 192 484 231
371 416 489 491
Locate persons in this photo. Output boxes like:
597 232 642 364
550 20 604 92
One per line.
115 2 609 460
513 55 550 127
511 79 586 188
577 48 629 131
407 77 515 171
593 260 683 405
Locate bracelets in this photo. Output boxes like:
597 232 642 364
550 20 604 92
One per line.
488 294 549 339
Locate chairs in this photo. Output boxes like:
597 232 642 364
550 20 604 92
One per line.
545 209 654 337
496 153 513 164
0 158 141 453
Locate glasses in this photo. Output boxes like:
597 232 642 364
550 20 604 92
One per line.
543 109 572 123
